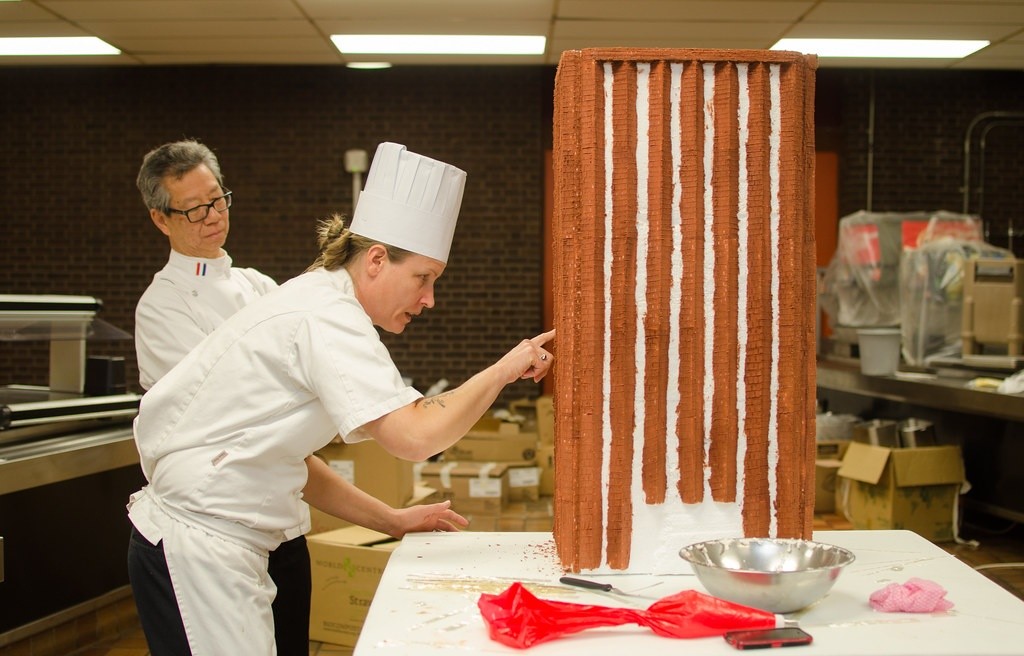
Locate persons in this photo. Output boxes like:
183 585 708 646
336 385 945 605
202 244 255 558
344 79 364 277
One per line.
124 142 555 656
132 141 311 656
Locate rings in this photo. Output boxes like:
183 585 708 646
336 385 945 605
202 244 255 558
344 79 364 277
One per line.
541 353 547 361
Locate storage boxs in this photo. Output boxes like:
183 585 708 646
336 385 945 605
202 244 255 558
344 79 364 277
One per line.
313 440 413 508
508 466 543 502
815 455 840 512
306 525 401 646
442 431 537 464
534 442 555 496
536 396 555 444
837 441 966 541
422 462 511 516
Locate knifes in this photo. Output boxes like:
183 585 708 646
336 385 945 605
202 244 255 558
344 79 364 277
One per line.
559 577 663 601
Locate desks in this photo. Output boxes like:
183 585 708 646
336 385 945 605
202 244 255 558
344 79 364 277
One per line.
351 530 1024 656
815 355 1024 422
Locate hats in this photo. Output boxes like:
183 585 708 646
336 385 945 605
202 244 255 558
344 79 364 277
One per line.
348 139 467 264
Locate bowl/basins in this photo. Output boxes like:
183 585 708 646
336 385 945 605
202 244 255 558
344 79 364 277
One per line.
678 538 855 614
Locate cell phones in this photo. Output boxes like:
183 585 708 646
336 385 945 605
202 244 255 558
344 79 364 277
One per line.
723 628 813 650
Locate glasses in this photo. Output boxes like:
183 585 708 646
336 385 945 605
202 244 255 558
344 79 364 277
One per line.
164 185 233 223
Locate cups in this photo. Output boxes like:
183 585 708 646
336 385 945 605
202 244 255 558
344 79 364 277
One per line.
857 329 901 376
